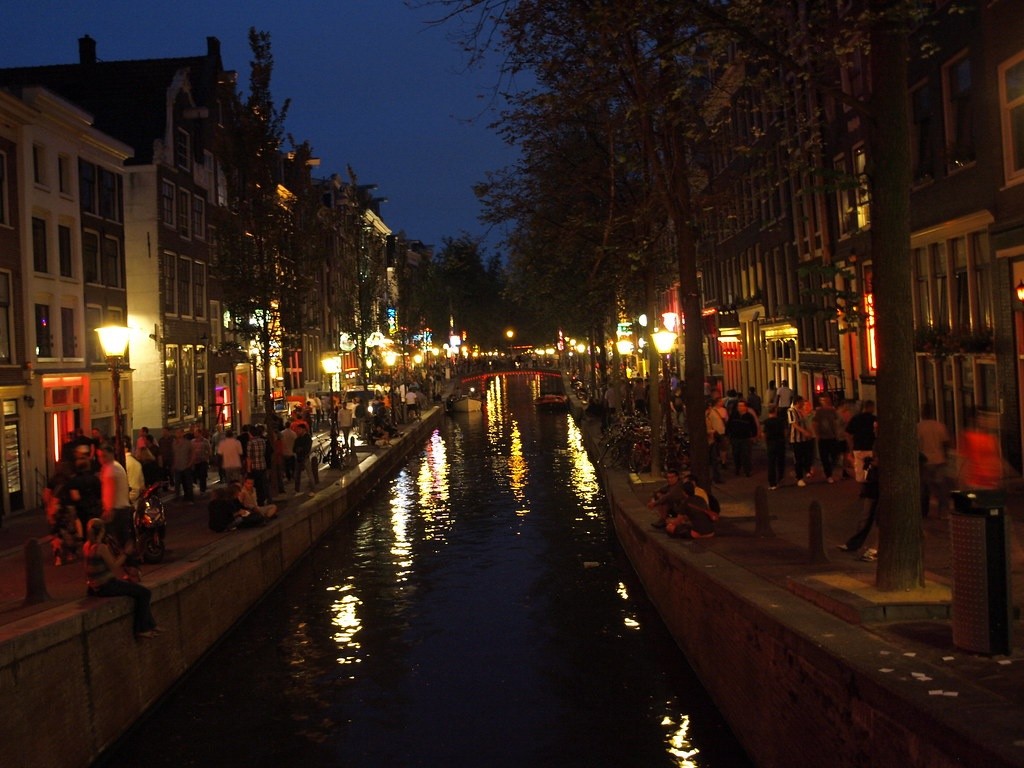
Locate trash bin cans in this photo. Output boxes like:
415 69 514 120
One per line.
948 488 1014 653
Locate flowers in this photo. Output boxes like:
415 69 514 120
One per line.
912 318 994 362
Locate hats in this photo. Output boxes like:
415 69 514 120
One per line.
78 445 90 454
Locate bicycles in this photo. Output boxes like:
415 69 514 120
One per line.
597 408 691 471
309 434 343 466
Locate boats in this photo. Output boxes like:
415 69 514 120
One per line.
533 394 569 412
451 395 482 412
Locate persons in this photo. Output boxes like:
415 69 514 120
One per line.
43 406 317 566
837 401 1024 575
307 367 435 448
646 469 720 539
425 355 625 407
603 372 840 490
82 518 167 639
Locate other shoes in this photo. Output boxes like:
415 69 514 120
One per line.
136 627 167 639
294 492 304 497
798 480 806 487
768 487 778 490
842 470 849 480
806 467 815 478
308 492 315 496
651 523 666 530
827 476 834 483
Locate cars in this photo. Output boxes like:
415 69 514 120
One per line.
341 385 384 426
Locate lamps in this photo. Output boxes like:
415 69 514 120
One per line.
1017 279 1024 301
201 333 209 353
23 394 35 408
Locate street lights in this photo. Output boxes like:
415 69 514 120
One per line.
384 351 398 426
94 321 131 471
322 357 342 468
653 329 677 456
617 340 634 416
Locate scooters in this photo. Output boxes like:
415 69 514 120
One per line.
133 479 173 565
